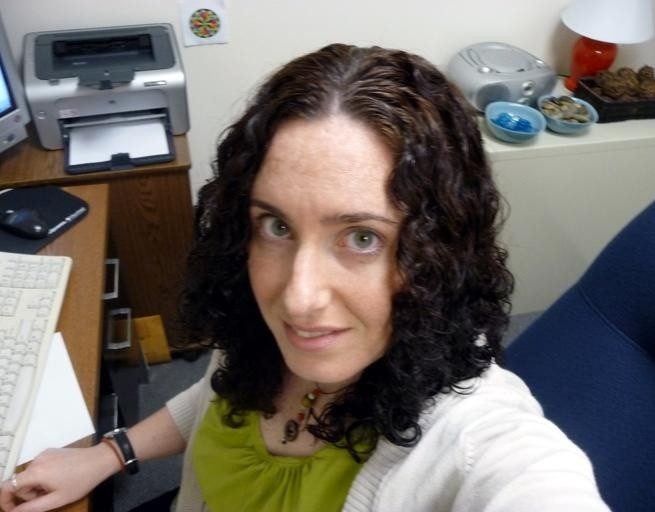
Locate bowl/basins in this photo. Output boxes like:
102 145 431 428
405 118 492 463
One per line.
537 93 599 134
485 101 547 143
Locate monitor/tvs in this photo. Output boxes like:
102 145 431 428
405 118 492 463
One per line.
0 33 31 156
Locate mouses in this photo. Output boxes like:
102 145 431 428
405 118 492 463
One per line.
0 204 50 236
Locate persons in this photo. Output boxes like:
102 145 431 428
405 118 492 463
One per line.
0 42 613 512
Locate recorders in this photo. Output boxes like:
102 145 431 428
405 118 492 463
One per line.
445 42 559 116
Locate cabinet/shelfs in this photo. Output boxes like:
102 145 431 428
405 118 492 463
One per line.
471 105 655 318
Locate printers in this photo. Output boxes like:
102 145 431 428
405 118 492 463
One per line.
22 22 191 173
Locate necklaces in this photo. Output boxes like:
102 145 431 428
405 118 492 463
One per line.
280 382 358 445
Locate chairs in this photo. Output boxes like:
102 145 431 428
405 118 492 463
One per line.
492 192 655 512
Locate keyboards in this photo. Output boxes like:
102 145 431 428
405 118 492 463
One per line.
1 251 73 483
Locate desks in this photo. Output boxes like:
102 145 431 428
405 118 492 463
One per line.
0 181 144 511
0 109 205 366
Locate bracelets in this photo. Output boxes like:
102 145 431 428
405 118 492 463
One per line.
98 437 125 474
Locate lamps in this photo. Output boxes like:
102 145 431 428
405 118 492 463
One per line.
558 2 655 92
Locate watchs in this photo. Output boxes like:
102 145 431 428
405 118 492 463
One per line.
104 427 140 476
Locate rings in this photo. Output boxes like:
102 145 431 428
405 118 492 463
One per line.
8 474 20 493
